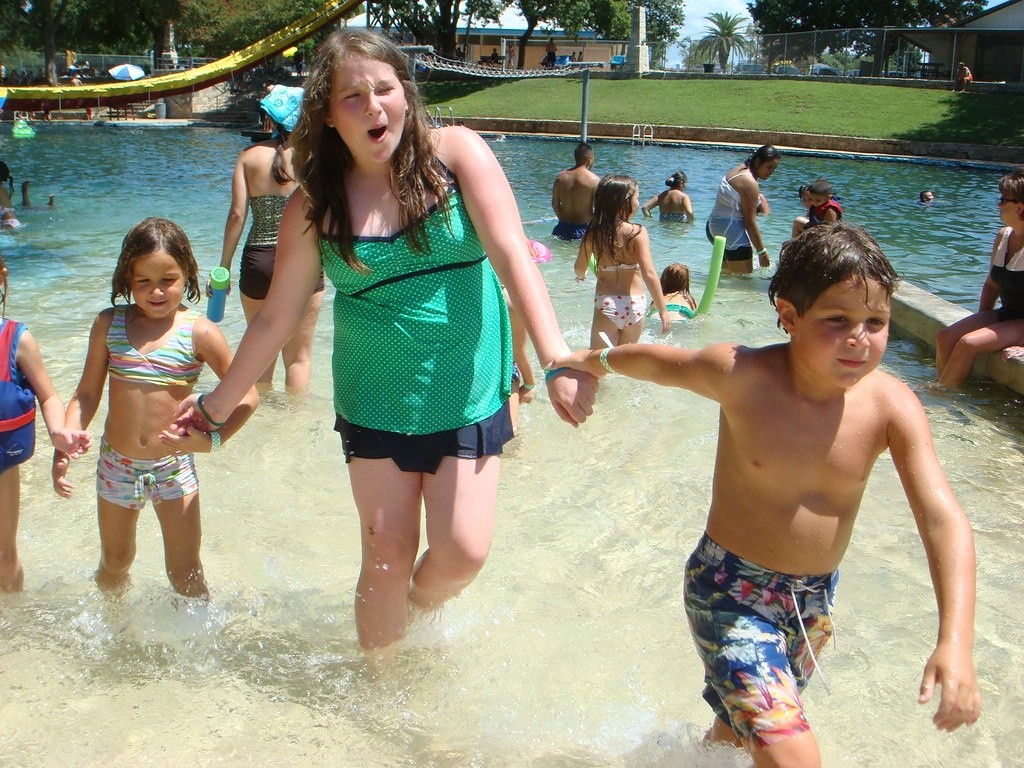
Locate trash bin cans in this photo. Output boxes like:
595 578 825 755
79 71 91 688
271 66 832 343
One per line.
155 102 167 118
703 64 715 73
860 60 874 76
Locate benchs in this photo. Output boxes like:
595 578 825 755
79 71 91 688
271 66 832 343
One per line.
478 56 506 68
566 62 607 68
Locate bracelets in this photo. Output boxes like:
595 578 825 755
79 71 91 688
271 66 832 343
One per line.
598 331 623 378
757 247 766 255
197 393 225 426
208 430 222 456
523 382 536 389
545 366 570 385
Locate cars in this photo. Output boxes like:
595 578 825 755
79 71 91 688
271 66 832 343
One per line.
609 55 627 71
776 66 802 75
809 68 842 75
845 69 906 77
555 54 574 69
741 65 766 74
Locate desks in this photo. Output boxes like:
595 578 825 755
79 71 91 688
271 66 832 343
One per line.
609 55 627 71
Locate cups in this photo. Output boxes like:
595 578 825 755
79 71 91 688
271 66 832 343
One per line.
207 267 231 322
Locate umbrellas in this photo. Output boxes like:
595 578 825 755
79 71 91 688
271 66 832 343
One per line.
108 64 145 81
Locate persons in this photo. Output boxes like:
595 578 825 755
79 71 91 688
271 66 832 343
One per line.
951 61 973 95
205 84 325 391
0 258 91 596
292 46 303 78
499 285 536 438
573 174 672 352
0 161 56 230
642 170 694 226
167 27 600 652
43 101 51 121
935 169 1024 391
919 190 934 203
457 48 463 57
646 263 698 323
551 143 601 241
705 145 781 276
544 226 982 768
539 38 558 70
86 104 93 120
62 57 102 86
791 179 842 239
0 61 44 87
570 52 583 69
52 215 260 597
488 43 516 69
